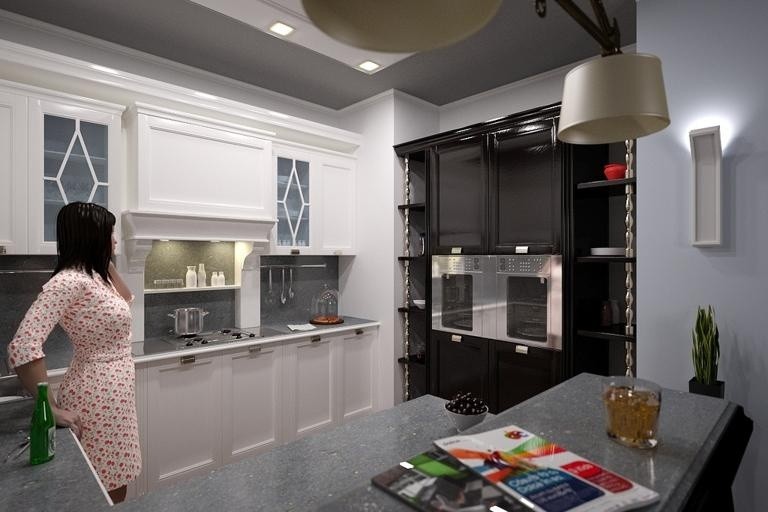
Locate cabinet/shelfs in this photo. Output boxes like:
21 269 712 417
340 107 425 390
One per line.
429 117 562 254
435 334 563 415
569 139 635 380
143 344 285 494
397 148 430 403
0 91 122 255
289 327 379 444
271 141 357 254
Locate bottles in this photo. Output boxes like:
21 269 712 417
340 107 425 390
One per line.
608 296 620 324
600 301 612 327
217 272 225 287
211 272 218 286
29 382 55 466
198 264 207 287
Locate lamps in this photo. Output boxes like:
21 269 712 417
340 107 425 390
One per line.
300 1 502 54
688 125 723 248
557 0 669 144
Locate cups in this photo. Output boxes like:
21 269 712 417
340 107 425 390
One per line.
602 377 662 449
153 279 184 289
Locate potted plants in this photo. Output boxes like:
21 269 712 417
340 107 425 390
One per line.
688 304 725 399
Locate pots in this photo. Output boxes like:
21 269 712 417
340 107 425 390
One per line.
167 308 209 335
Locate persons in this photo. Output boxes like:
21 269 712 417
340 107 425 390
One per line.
7 202 142 504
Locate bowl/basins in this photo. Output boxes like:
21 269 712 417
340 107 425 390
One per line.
444 401 489 431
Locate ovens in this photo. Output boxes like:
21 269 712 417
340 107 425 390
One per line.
431 255 562 350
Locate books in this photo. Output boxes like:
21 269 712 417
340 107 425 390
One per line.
432 424 660 511
370 446 536 512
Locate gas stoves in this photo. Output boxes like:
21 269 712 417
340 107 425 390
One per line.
161 327 256 347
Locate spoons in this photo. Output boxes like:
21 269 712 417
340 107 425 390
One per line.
289 267 295 299
281 268 288 304
267 267 276 304
